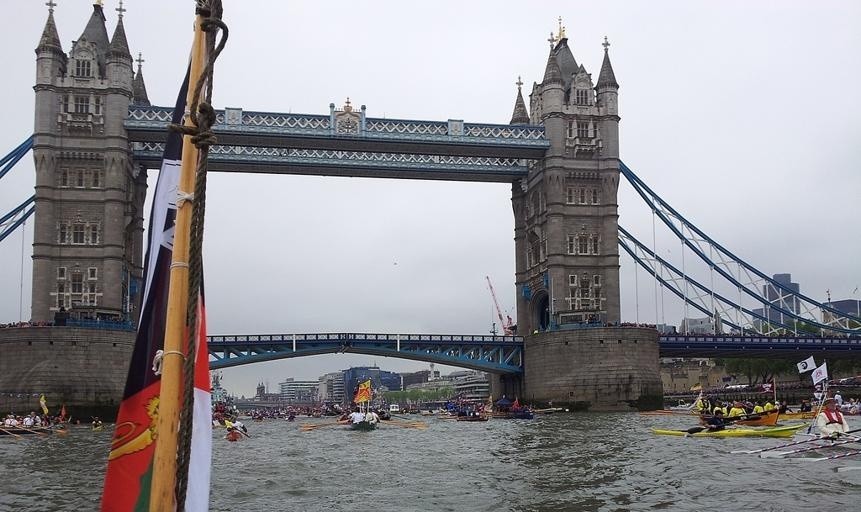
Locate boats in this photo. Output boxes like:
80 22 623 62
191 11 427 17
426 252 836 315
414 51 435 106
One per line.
1 395 104 435
640 356 860 488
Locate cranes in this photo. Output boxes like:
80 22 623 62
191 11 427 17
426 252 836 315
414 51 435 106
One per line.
486 275 512 335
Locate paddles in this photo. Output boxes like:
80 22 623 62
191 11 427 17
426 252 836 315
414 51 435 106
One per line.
729 433 861 460
639 410 732 419
1 423 68 440
298 417 351 431
376 419 430 429
688 417 761 434
435 410 458 419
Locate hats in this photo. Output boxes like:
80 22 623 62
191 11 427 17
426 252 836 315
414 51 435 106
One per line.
715 410 725 415
821 390 835 406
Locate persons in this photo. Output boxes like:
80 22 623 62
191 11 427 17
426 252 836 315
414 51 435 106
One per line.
212 399 391 439
0 320 51 327
446 399 532 417
694 390 861 438
621 322 849 338
1 411 103 429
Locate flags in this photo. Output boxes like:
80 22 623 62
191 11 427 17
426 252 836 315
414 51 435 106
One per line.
811 362 828 386
100 24 212 512
353 379 371 404
797 355 817 374
